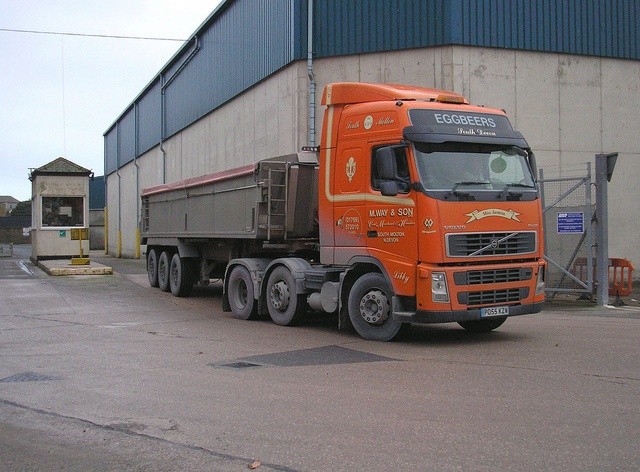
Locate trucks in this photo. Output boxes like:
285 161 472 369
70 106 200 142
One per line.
141 83 548 341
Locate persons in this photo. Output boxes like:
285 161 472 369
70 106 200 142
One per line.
461 159 492 190
45 207 68 226
